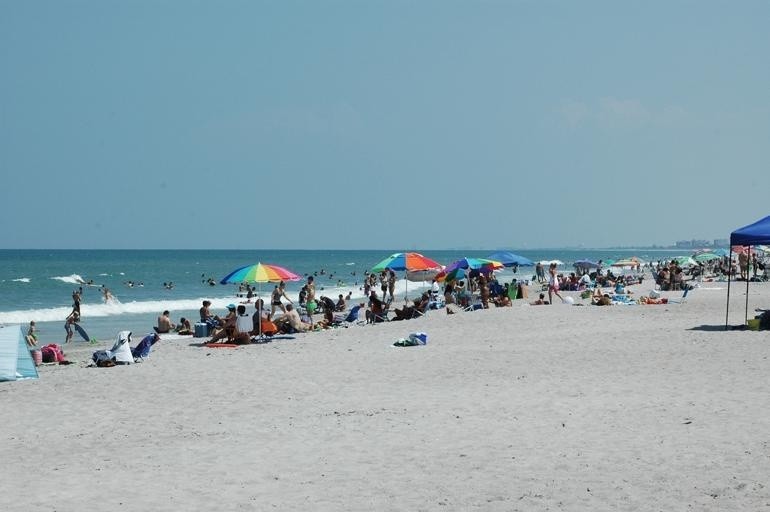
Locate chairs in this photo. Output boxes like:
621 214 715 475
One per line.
345 296 393 325
412 297 431 319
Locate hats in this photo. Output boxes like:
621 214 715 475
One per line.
226 304 235 309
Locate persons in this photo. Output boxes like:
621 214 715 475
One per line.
432 262 564 309
25 272 266 350
642 296 668 304
698 251 770 280
645 258 702 291
252 269 429 339
560 263 645 306
